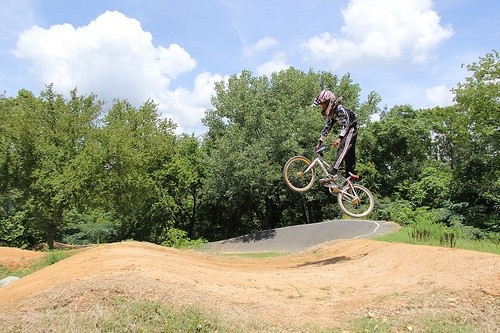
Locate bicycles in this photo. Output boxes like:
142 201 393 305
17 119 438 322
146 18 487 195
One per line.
283 142 375 217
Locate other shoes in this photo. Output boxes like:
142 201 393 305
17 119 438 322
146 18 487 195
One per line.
319 174 338 183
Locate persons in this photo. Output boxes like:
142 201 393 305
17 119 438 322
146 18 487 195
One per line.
312 88 358 183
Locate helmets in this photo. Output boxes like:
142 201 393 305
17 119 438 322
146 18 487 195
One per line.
312 89 337 116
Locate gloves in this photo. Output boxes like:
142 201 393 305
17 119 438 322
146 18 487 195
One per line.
332 138 342 147
314 139 324 151
